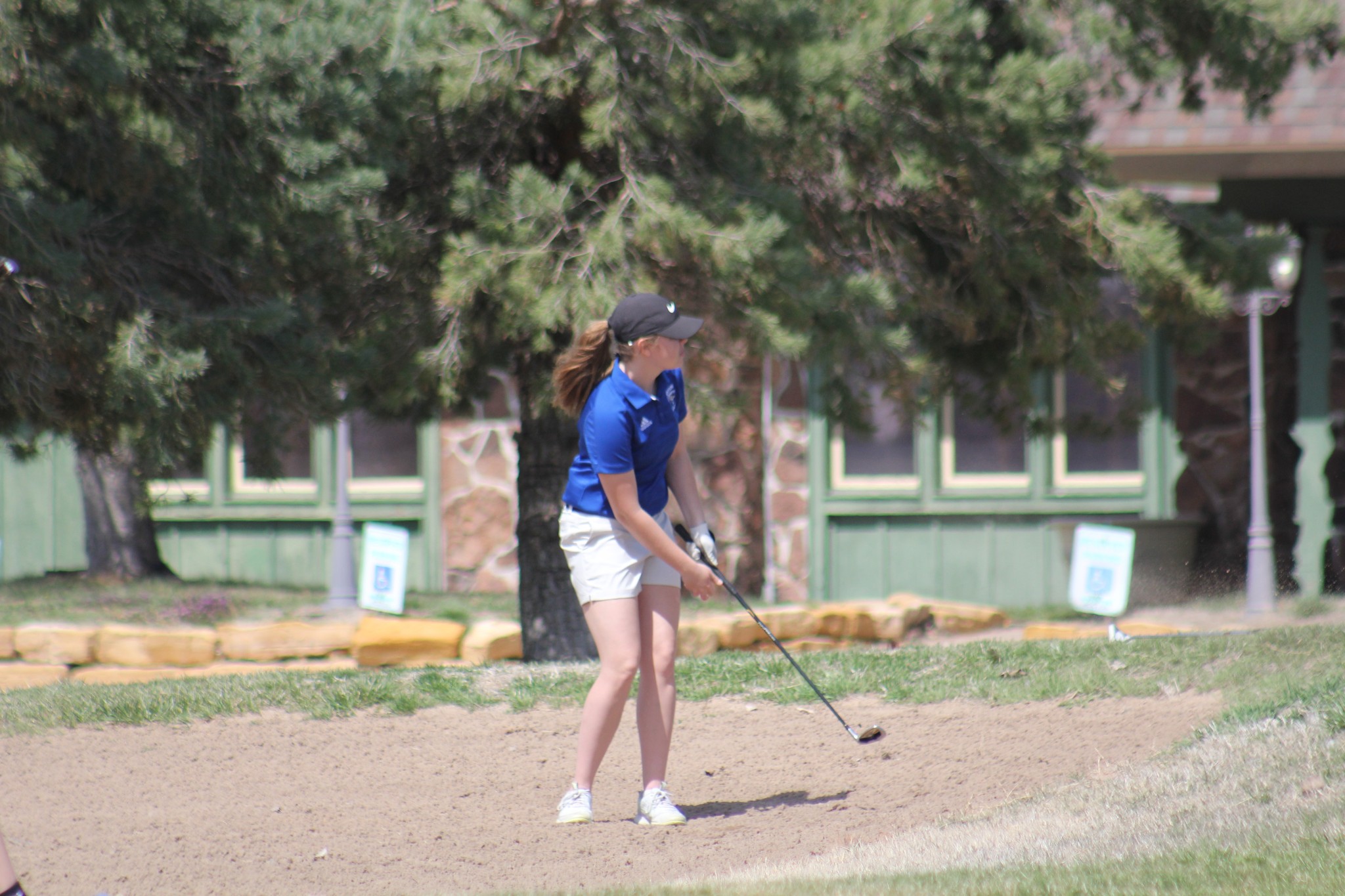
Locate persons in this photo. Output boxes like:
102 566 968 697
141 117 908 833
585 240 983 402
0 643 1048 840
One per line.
551 294 722 827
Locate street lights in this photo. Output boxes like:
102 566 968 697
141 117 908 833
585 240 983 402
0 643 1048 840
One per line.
1209 223 1302 618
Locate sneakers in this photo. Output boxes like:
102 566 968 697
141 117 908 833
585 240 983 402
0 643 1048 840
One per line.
633 782 686 826
557 781 594 824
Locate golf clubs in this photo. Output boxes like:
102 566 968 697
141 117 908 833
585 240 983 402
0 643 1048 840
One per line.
673 521 887 745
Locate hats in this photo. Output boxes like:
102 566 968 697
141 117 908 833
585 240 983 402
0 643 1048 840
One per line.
607 293 704 343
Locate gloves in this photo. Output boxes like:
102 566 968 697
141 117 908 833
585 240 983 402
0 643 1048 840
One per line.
687 521 719 566
